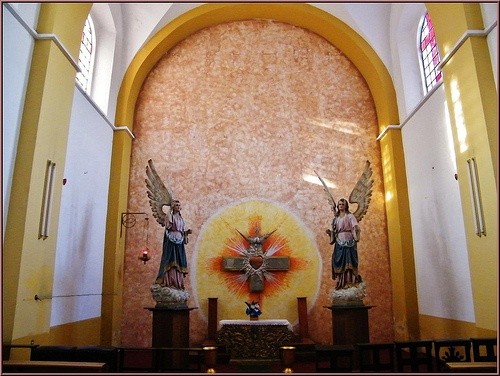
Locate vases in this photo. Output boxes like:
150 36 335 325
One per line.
250 315 258 321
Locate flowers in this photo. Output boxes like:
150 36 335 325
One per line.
245 301 262 316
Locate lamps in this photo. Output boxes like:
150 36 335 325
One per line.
121 212 151 264
467 158 486 237
38 160 56 240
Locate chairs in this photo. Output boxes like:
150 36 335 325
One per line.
316 337 498 373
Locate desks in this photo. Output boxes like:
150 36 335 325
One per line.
218 320 293 373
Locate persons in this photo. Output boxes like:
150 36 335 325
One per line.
326 199 362 289
155 200 192 291
